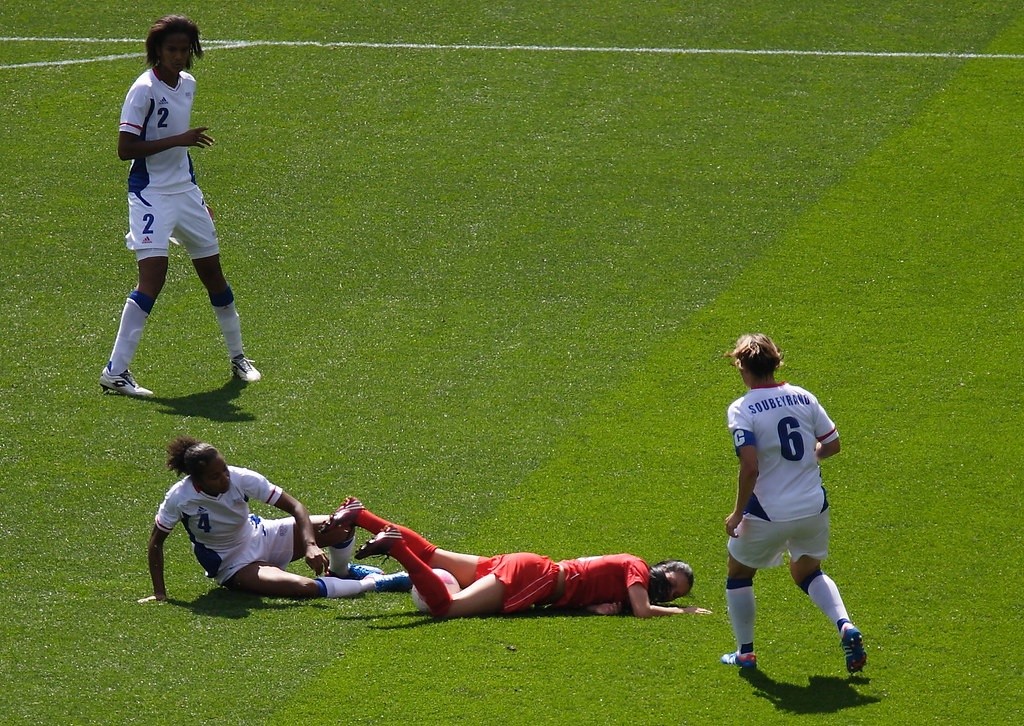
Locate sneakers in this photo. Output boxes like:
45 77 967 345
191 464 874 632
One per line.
722 649 756 668
329 562 384 580
100 367 153 398
318 496 367 535
231 354 261 382
839 627 867 675
354 523 404 564
363 571 413 594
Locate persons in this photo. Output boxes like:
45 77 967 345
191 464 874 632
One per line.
137 434 414 603
318 496 715 619
99 14 261 399
718 332 866 673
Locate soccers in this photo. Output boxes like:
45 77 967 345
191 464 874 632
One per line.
410 568 462 612
